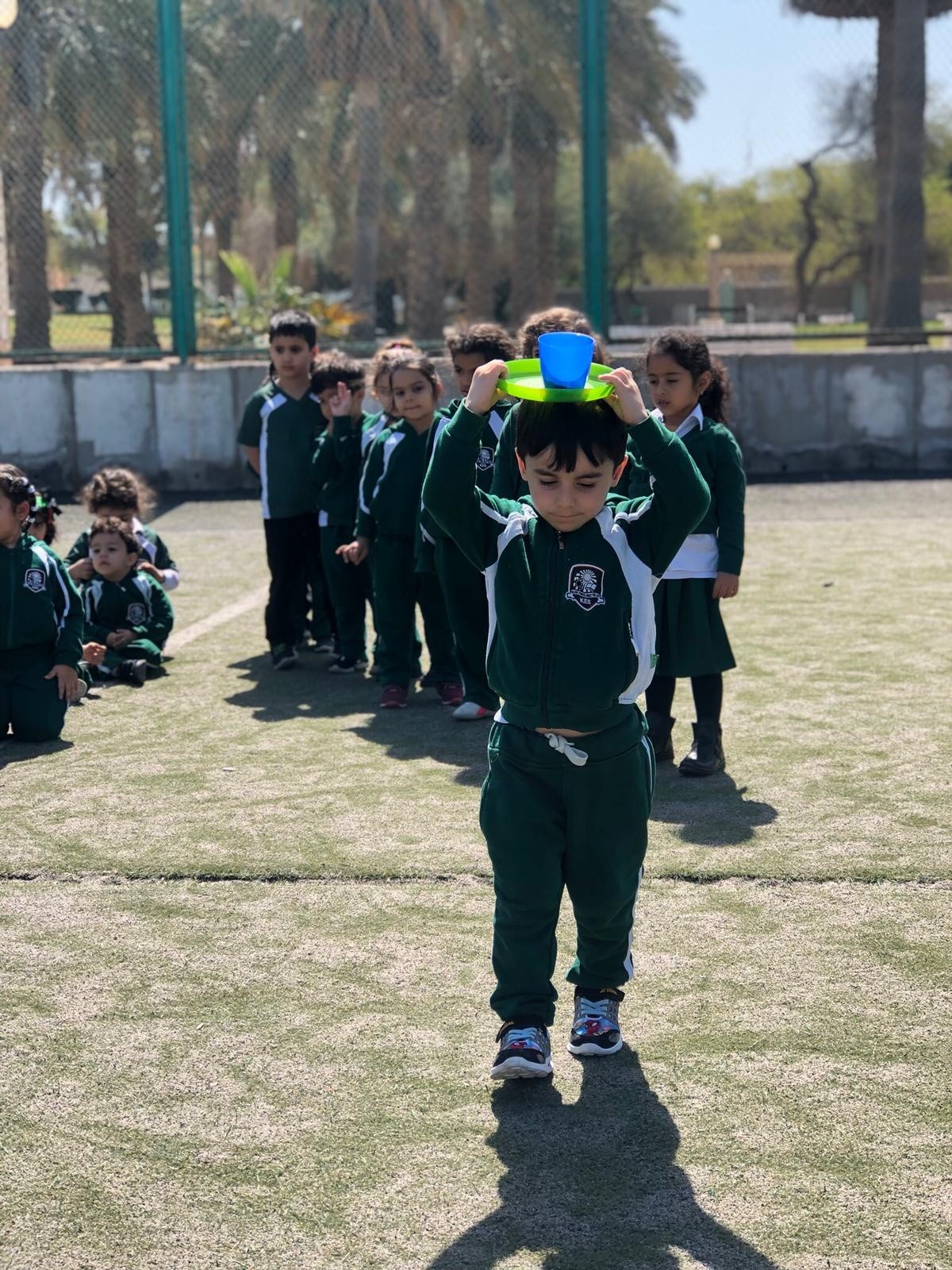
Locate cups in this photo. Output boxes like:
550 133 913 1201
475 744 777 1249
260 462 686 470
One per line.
537 331 595 388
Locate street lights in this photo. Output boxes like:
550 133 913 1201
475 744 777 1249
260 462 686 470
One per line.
706 234 722 319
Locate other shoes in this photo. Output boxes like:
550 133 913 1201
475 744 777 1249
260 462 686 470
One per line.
328 657 369 673
72 678 88 703
120 658 147 684
311 635 337 653
679 721 726 772
273 645 299 668
644 712 676 760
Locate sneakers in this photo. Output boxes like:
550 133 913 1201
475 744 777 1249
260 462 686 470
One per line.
453 702 495 719
379 685 406 708
568 984 625 1055
490 1017 552 1077
437 681 463 705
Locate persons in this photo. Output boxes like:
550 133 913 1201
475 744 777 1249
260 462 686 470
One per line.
239 308 613 719
77 516 174 685
421 360 712 1078
62 468 181 592
0 463 85 745
608 328 746 774
27 468 92 702
